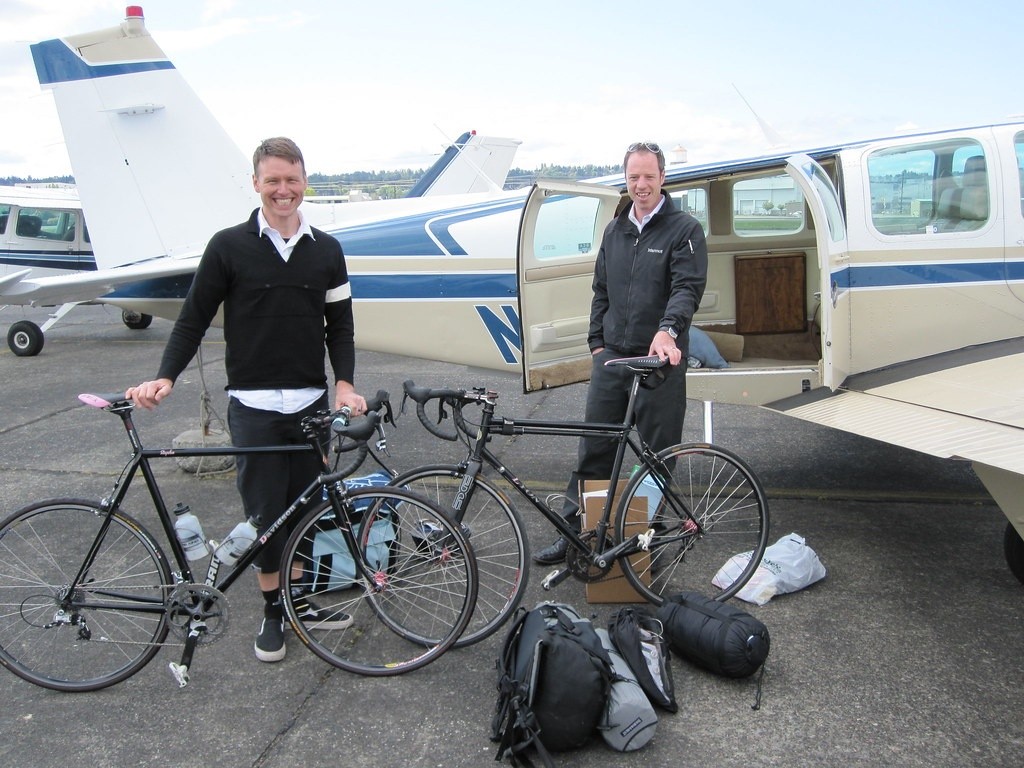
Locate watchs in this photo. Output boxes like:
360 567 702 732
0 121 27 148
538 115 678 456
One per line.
658 326 678 339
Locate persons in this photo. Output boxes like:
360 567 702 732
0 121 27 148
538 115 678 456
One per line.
126 137 367 662
532 143 708 564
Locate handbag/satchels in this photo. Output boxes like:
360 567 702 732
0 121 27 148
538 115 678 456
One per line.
712 533 826 604
312 471 408 593
608 607 679 714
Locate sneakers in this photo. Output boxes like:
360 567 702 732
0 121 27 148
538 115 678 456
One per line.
254 611 286 661
285 601 354 630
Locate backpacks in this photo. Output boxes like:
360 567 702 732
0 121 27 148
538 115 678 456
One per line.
491 600 613 760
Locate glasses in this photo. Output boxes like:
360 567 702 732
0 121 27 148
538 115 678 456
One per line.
628 141 660 153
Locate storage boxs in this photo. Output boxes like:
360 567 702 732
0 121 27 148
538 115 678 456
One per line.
573 466 666 603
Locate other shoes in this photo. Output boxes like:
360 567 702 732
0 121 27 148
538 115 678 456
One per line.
533 537 568 564
651 550 660 571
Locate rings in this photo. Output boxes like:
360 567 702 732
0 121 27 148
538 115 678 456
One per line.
671 348 675 350
358 409 361 412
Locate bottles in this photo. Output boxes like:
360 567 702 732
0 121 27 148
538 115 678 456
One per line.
214 513 263 568
172 501 210 561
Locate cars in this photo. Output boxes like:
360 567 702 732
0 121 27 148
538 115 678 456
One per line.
791 211 802 218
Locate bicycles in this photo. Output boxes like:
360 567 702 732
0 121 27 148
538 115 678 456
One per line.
1 390 480 694
355 352 770 653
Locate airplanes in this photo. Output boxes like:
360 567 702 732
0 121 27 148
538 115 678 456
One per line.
23 5 1024 586
0 133 523 356
